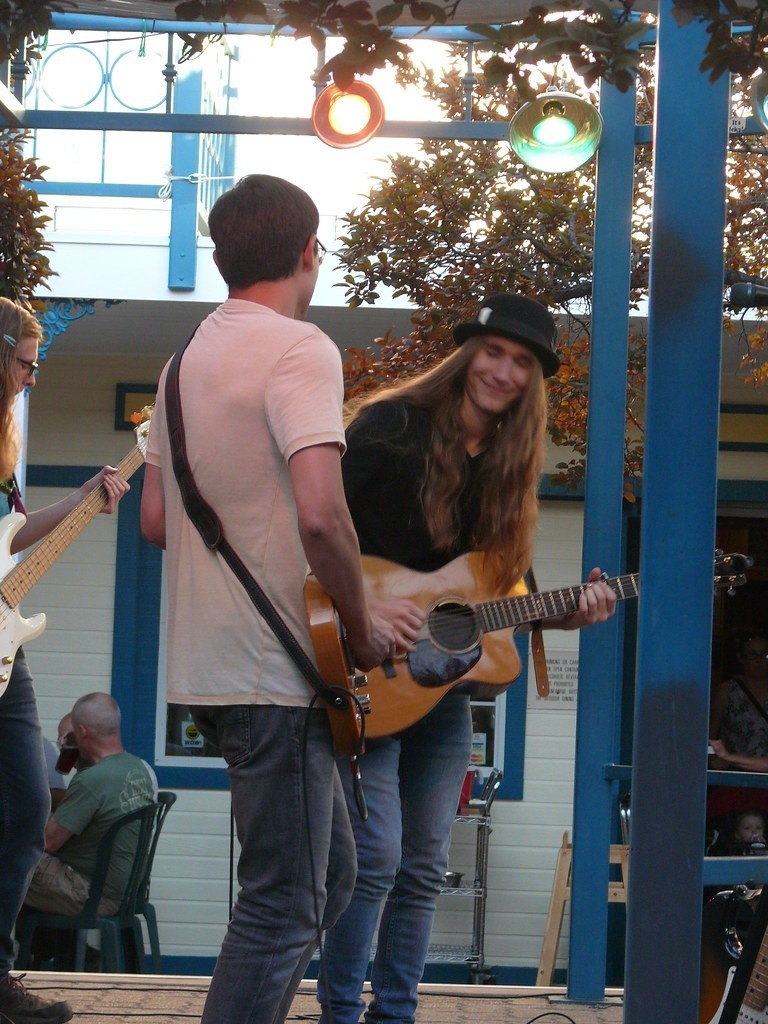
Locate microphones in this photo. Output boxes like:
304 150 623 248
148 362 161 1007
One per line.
730 282 768 308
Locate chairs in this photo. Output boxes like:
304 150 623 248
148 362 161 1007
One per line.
18 790 179 976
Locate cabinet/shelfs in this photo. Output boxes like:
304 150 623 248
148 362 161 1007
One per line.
367 765 502 984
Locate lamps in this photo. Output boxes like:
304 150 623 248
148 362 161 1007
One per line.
310 81 386 150
506 68 604 174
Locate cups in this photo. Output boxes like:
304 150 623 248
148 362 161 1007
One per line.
54 732 80 776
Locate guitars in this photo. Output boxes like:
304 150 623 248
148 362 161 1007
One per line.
302 575 365 761
0 401 154 698
360 548 754 741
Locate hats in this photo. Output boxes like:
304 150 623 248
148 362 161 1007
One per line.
453 292 561 379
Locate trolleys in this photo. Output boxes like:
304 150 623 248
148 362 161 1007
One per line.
311 767 497 985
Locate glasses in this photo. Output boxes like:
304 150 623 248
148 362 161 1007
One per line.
14 356 39 377
741 650 768 660
314 236 328 265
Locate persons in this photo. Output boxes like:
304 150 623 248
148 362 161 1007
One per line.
0 299 130 1024
710 620 768 773
20 692 160 973
730 807 766 858
135 173 397 1024
318 294 620 1024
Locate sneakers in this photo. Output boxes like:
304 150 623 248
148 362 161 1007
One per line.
0 974 76 1024
